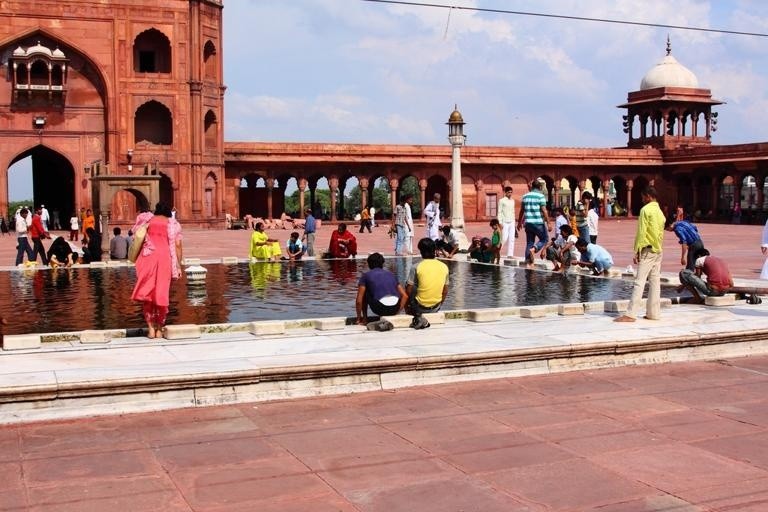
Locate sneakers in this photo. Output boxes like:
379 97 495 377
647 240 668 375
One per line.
409 316 430 329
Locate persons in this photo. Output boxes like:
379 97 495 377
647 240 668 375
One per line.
329 260 357 287
676 249 735 304
405 236 450 329
126 201 155 237
353 253 409 326
390 195 411 256
404 194 417 255
249 222 281 260
662 215 704 271
467 179 627 275
358 205 372 233
328 222 357 258
612 185 668 323
125 228 134 246
248 263 281 300
299 208 316 256
438 226 460 258
285 232 306 260
284 261 303 289
759 216 768 281
368 204 375 228
13 204 108 266
422 193 444 241
131 200 182 339
109 227 128 259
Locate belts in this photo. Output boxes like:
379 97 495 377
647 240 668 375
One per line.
414 297 442 310
643 245 652 249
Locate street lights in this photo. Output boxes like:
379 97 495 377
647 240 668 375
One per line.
443 99 469 253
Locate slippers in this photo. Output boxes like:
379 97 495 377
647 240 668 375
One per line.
367 320 394 331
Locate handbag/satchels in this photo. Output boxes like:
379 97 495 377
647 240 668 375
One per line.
127 223 150 264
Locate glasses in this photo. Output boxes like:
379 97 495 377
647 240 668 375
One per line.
506 191 512 194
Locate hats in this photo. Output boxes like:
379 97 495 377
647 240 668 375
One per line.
19 205 45 208
